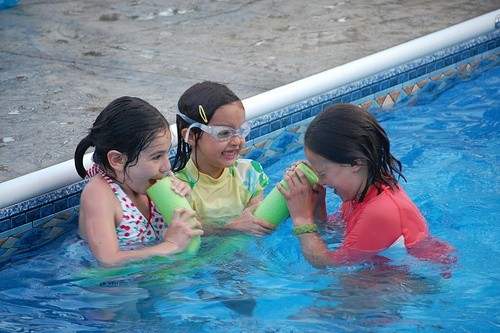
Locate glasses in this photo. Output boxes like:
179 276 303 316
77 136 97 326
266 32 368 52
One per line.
176 105 250 142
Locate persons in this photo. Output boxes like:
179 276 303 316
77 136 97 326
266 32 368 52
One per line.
276 103 456 326
172 80 277 238
64 96 204 321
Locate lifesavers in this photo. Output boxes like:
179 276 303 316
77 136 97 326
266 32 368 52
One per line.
146 177 200 251
252 164 319 227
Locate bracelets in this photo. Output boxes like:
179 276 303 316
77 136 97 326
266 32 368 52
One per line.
293 223 319 236
